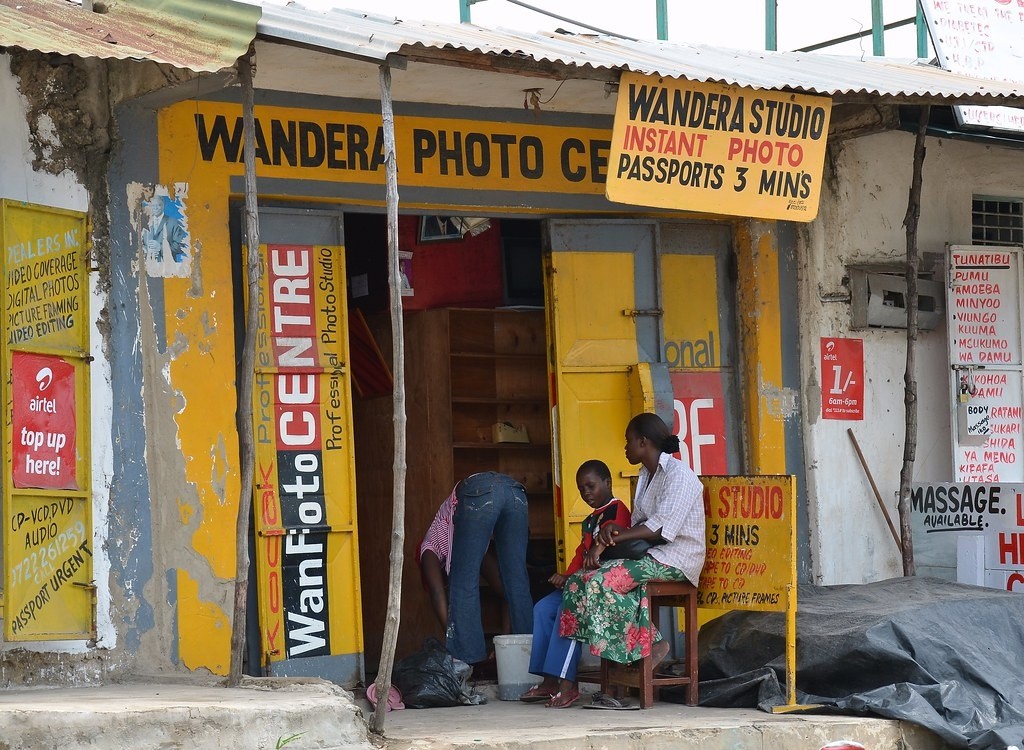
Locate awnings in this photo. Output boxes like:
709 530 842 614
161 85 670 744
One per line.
0 0 262 690
240 0 1024 739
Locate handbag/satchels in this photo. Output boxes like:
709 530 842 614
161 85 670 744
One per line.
599 520 667 560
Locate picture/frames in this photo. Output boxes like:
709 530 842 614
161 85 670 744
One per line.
415 216 465 246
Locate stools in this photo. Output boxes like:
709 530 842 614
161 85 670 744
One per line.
577 579 700 707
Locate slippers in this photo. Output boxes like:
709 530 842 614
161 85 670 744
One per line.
545 692 580 709
582 691 641 711
520 685 552 702
388 685 405 710
367 683 391 712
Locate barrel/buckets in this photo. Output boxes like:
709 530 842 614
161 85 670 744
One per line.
493 634 544 701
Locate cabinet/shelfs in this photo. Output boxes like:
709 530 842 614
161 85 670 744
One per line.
423 306 555 632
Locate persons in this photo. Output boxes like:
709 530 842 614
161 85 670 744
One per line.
520 459 632 708
560 411 708 675
418 471 534 690
